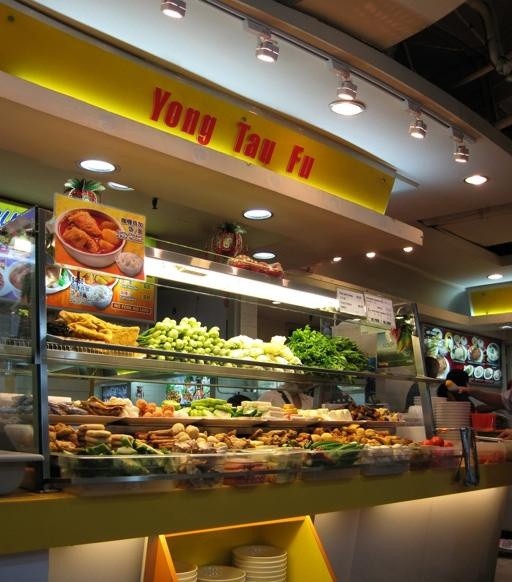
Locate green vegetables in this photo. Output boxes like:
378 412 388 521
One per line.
287 324 370 373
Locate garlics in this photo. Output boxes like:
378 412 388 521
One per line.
351 444 413 465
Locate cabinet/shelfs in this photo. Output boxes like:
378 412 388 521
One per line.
0 206 512 581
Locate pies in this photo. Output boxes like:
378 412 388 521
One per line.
77 423 134 452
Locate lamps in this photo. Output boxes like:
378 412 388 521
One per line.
161 0 470 163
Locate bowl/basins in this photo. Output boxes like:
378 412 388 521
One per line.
5 424 33 451
89 295 112 311
116 262 142 277
55 207 126 269
176 544 288 582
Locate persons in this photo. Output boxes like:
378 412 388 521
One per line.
255 380 314 411
405 357 512 440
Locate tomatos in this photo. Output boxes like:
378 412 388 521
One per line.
422 436 460 468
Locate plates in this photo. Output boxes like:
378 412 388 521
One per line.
261 417 291 428
475 436 503 442
464 365 502 381
200 417 269 428
20 414 126 428
431 328 484 363
413 396 471 428
316 420 368 428
291 417 322 427
45 269 74 295
108 417 204 428
367 420 407 429
486 343 501 361
68 269 118 288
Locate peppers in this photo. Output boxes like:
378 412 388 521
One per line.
304 441 364 468
